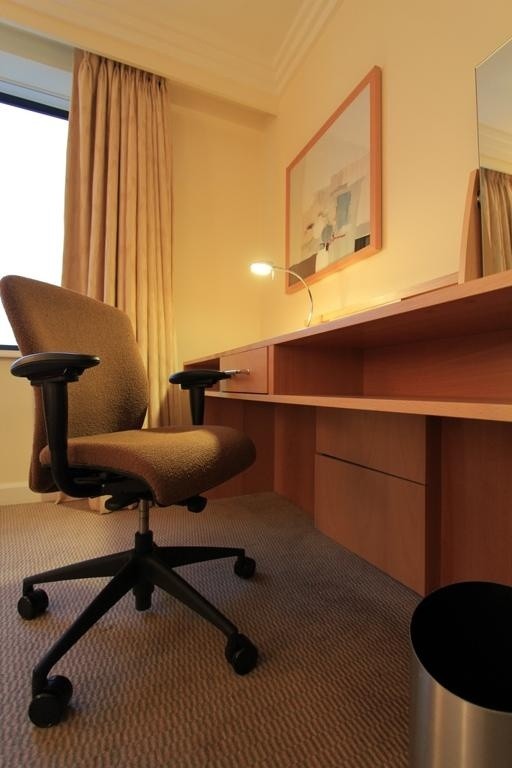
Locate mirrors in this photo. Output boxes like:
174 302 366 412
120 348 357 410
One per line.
473 35 512 277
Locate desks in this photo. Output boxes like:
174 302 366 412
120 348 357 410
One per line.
181 268 512 598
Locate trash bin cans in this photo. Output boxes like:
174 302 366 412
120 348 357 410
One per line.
408 580 512 768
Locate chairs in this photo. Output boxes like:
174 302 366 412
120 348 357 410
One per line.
0 273 259 729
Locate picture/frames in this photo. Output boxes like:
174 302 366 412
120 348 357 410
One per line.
283 65 384 295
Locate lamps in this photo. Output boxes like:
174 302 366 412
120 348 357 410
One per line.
250 260 314 328
